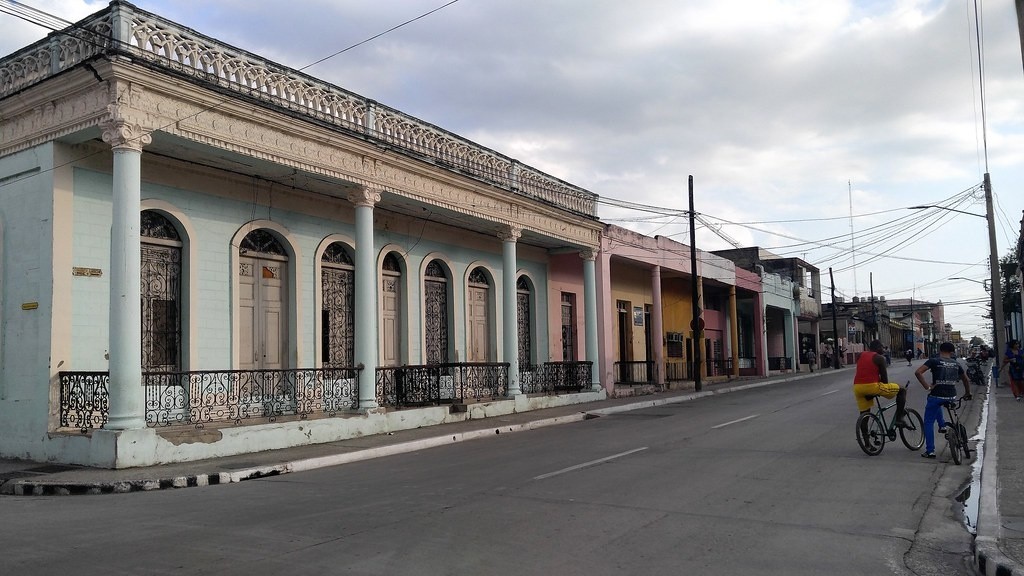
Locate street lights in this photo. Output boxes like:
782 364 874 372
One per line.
906 173 1010 388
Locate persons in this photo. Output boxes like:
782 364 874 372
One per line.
916 348 923 359
854 340 907 449
906 347 913 367
838 345 848 369
915 342 971 459
806 348 816 373
997 340 1024 401
883 345 891 368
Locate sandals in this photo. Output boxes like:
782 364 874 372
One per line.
865 445 877 451
894 420 915 430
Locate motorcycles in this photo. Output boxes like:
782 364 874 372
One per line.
962 354 985 385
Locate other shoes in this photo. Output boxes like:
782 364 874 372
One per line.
921 452 936 458
938 427 947 433
1017 397 1020 400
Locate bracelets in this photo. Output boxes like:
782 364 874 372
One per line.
925 386 931 392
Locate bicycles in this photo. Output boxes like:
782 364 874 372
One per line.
927 385 972 465
854 380 925 456
